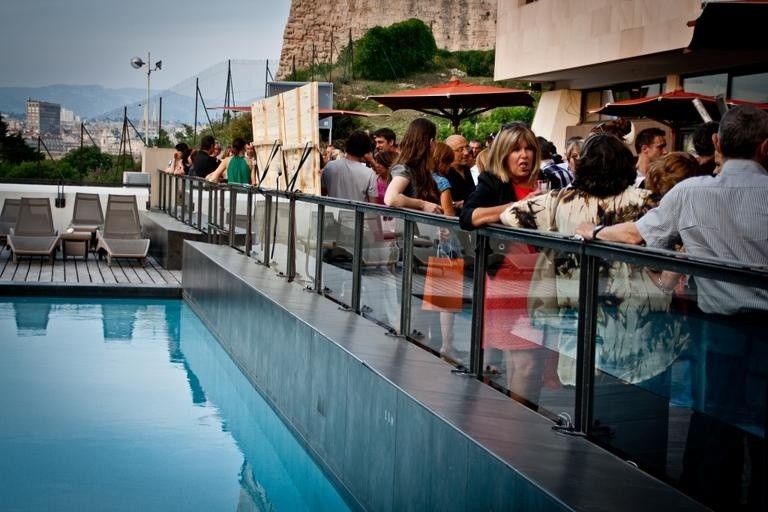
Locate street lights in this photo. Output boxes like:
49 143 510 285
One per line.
128 48 163 147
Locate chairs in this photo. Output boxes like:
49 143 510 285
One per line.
0 193 152 269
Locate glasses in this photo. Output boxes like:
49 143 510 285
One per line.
452 146 472 153
596 122 605 132
502 123 531 133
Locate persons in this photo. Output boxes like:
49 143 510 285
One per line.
320 106 768 512
166 135 259 187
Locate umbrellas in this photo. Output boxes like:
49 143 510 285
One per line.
585 85 767 152
368 74 536 133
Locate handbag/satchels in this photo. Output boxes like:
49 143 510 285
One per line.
421 254 465 313
